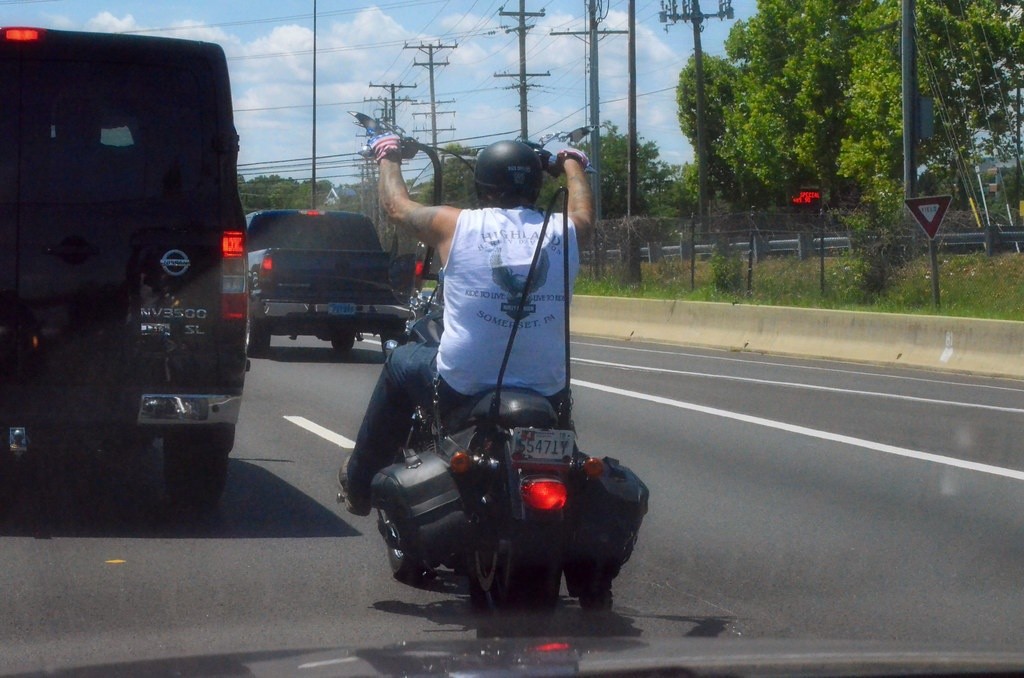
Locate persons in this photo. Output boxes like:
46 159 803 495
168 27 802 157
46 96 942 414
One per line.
340 126 594 518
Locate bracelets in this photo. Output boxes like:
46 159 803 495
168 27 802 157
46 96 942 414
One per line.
562 151 583 163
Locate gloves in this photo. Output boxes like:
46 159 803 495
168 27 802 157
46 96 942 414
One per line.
557 148 589 168
367 130 402 165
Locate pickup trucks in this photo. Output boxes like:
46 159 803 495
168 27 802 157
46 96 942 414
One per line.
243 209 414 349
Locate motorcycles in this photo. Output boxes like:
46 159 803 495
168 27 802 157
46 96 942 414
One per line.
337 114 647 614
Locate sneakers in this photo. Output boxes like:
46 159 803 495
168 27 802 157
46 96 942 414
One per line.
337 454 371 516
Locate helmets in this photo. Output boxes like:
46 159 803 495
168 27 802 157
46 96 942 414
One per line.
473 140 544 187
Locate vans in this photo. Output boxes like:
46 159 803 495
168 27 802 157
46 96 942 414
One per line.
1 27 249 491
